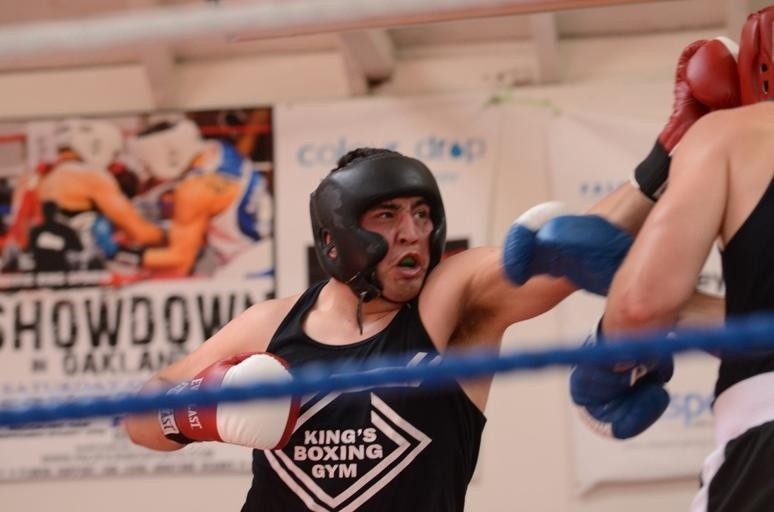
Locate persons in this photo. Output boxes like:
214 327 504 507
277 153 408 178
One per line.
42 131 159 273
501 1 773 511
132 112 275 274
127 32 741 512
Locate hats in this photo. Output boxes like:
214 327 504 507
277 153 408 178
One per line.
306 152 452 301
737 7 774 102
55 116 204 179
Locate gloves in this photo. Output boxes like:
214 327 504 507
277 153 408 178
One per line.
72 156 176 266
157 355 301 453
628 37 739 203
567 310 674 440
500 200 634 297
